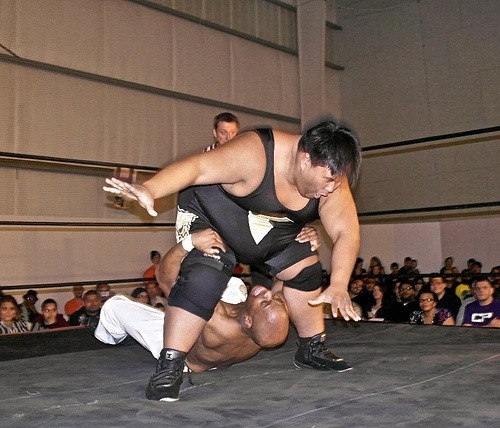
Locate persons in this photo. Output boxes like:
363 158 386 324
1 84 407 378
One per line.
103 120 362 404
349 256 500 327
0 283 166 334
175 110 241 243
86 225 322 371
142 250 161 280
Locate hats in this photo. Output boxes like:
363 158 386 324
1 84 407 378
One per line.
64 298 86 316
23 290 38 300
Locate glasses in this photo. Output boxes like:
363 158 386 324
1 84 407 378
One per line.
419 298 434 302
43 309 56 313
400 286 413 292
25 298 34 301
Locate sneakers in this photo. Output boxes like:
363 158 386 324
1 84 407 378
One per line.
145 348 188 402
292 330 354 372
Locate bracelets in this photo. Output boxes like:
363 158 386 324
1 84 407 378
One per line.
182 234 194 252
